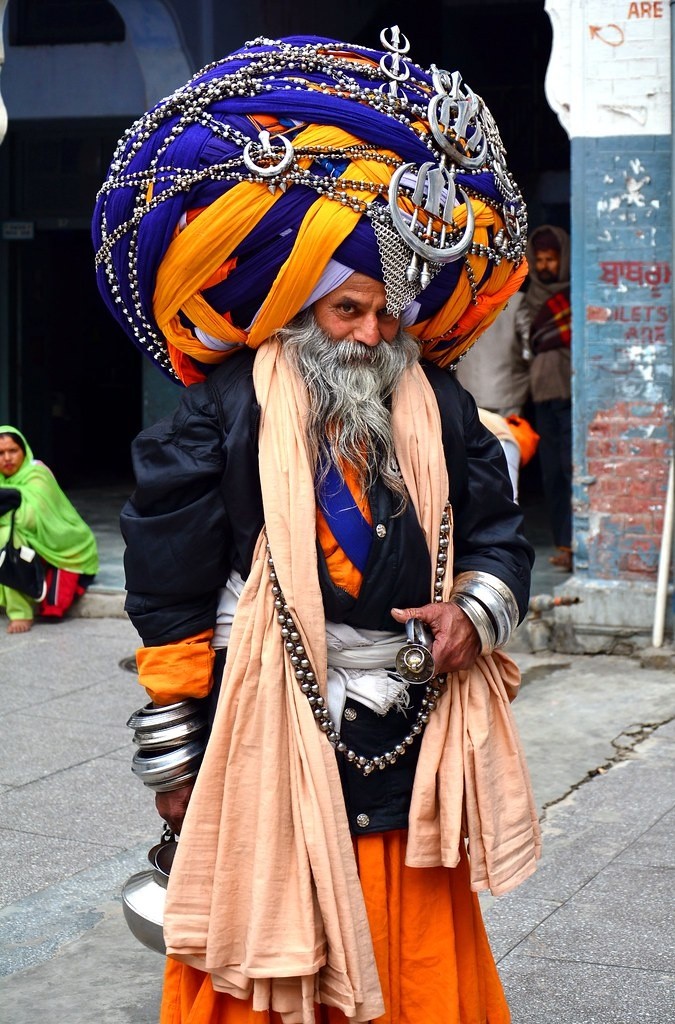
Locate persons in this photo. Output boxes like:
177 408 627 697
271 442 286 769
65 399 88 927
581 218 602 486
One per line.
121 219 541 1024
0 424 100 634
455 224 572 570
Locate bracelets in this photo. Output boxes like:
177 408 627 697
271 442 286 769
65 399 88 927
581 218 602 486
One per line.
125 699 211 792
450 570 519 656
